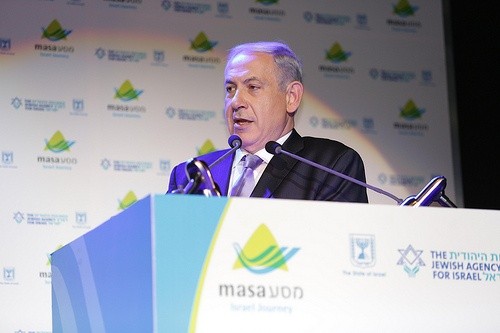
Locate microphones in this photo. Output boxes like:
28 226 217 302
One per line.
264 142 447 206
180 135 243 194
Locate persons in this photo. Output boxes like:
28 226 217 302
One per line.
166 42 368 203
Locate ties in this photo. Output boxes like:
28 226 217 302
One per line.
231 155 263 197
